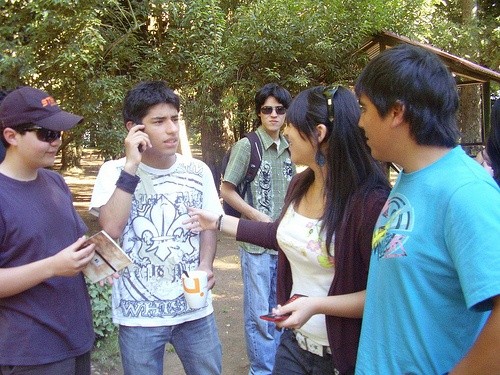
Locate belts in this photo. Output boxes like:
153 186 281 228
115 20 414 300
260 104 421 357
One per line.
293 330 332 357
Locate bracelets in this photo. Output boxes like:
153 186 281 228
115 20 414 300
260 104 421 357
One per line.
216 214 224 231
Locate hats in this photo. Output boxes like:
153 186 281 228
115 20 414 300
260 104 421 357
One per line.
0 87 84 131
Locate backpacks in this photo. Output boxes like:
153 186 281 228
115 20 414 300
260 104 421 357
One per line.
221 131 262 218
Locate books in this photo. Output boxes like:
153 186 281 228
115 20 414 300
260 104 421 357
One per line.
80 231 134 284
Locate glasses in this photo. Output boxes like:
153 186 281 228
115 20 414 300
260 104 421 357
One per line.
260 106 287 115
16 128 61 143
323 85 341 142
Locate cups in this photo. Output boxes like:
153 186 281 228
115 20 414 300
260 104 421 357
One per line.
181 270 208 309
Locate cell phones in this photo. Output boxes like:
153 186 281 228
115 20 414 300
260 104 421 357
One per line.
260 293 309 322
136 123 144 152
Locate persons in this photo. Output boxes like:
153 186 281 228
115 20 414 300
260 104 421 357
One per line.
357 45 500 375
0 87 119 375
89 84 225 375
181 86 392 375
476 98 500 188
220 83 299 375
